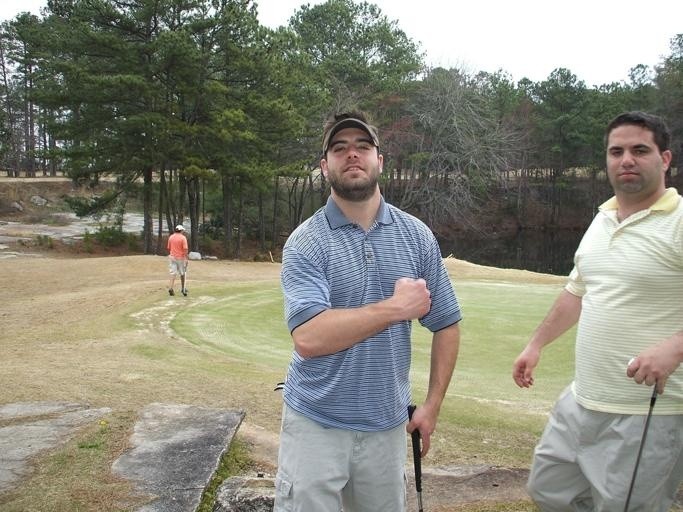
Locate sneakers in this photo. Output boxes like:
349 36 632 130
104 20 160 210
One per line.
168 288 188 296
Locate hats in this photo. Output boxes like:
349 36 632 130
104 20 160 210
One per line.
176 225 185 231
322 118 379 156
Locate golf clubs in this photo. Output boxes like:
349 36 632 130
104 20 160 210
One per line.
183 267 188 296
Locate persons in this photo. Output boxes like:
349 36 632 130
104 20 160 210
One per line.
272 108 462 512
166 225 188 296
510 110 683 512
